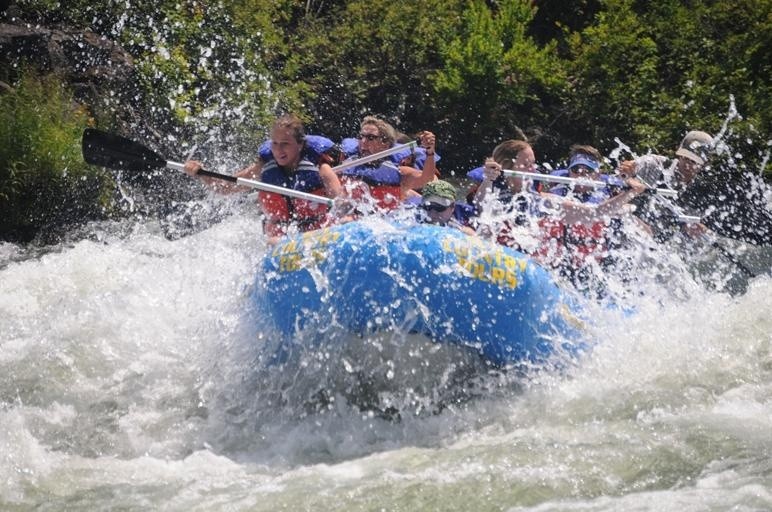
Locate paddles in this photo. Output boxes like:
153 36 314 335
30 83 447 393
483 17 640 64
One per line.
667 207 772 246
677 165 748 209
83 127 359 211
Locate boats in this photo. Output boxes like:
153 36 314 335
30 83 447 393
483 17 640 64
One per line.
262 215 646 377
682 237 771 297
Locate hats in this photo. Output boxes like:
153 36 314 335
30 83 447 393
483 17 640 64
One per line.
419 180 457 207
567 153 600 174
676 131 715 166
